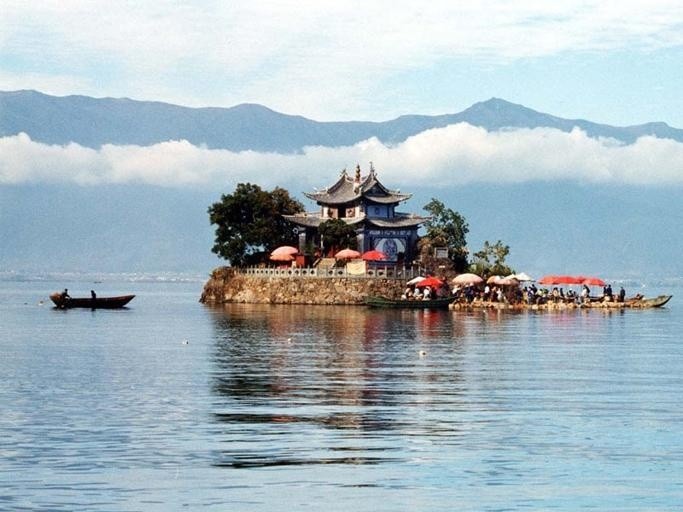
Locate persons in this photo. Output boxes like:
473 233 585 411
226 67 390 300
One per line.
61 288 69 299
402 272 627 305
90 290 95 299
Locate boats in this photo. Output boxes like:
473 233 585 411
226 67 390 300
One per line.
583 295 673 307
361 295 458 309
49 295 136 308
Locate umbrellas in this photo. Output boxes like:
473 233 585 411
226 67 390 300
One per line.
266 243 389 270
